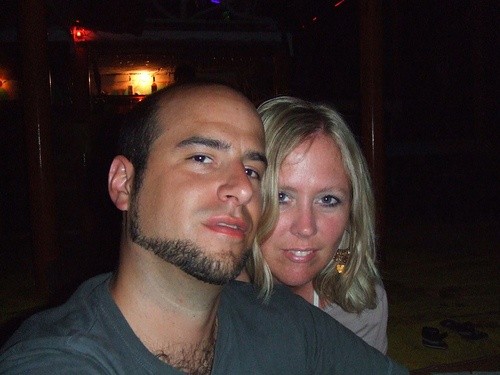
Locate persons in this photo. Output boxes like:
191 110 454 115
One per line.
235 96 389 358
0 80 409 375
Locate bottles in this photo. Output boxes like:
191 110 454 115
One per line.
127 73 133 95
152 77 157 93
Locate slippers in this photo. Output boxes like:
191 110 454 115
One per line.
420 325 449 350
439 319 488 339
